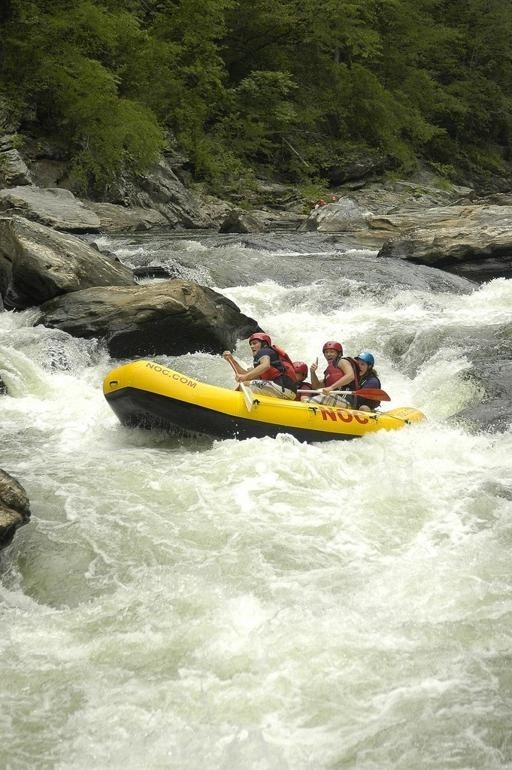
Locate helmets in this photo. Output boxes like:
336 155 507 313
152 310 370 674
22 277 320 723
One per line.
249 333 272 347
355 352 375 366
323 341 344 355
294 361 308 375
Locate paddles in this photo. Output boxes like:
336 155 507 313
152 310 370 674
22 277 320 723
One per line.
297 388 391 401
227 355 254 412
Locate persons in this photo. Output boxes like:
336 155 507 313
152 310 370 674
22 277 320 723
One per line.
353 352 382 413
221 332 298 400
290 361 313 402
303 340 360 410
314 199 327 210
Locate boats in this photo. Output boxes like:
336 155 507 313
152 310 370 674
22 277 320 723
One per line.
102 360 429 440
